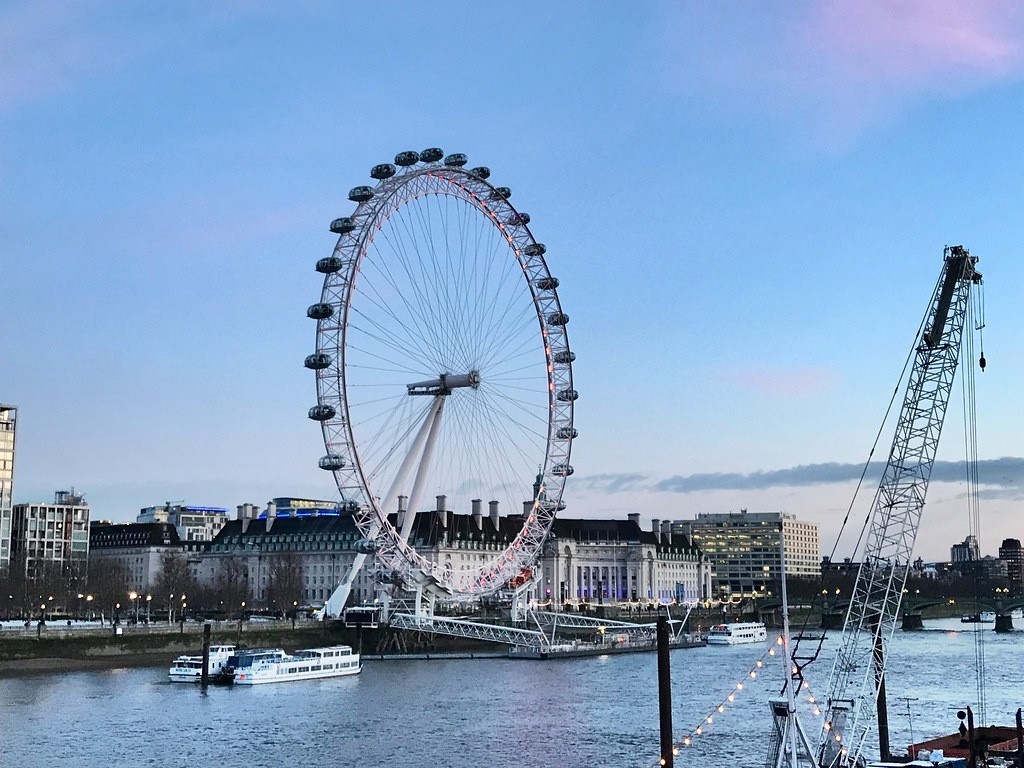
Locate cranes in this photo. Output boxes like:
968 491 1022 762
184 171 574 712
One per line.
813 243 988 768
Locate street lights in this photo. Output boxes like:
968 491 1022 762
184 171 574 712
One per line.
518 591 772 619
916 590 919 599
1003 588 1010 599
325 601 328 617
77 594 93 622
115 603 121 624
903 589 909 598
39 604 46 625
995 588 1001 600
242 601 246 620
143 596 152 624
292 601 297 630
183 603 186 622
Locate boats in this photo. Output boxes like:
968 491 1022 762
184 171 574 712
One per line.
961 614 980 622
707 622 767 644
168 645 236 682
1011 610 1022 619
233 644 363 685
791 636 829 641
980 611 996 623
